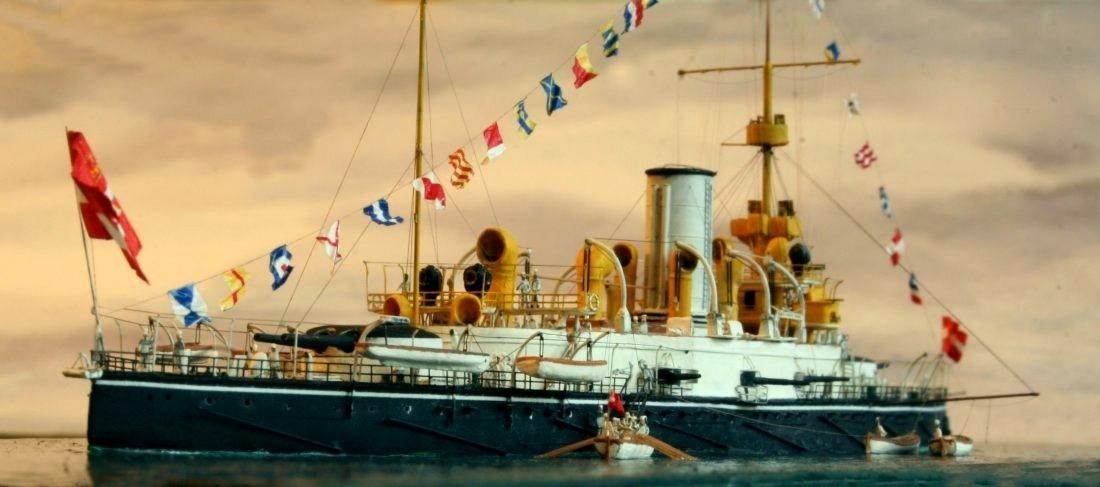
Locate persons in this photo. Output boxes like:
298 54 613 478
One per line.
875 417 888 439
933 419 943 438
608 388 625 417
594 405 649 441
171 328 185 365
267 344 281 378
134 331 155 371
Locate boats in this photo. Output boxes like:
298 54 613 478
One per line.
593 438 655 459
865 431 974 457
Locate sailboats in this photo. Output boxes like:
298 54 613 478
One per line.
59 0 1041 464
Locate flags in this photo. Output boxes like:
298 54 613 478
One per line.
810 0 929 305
68 130 153 286
939 313 970 365
